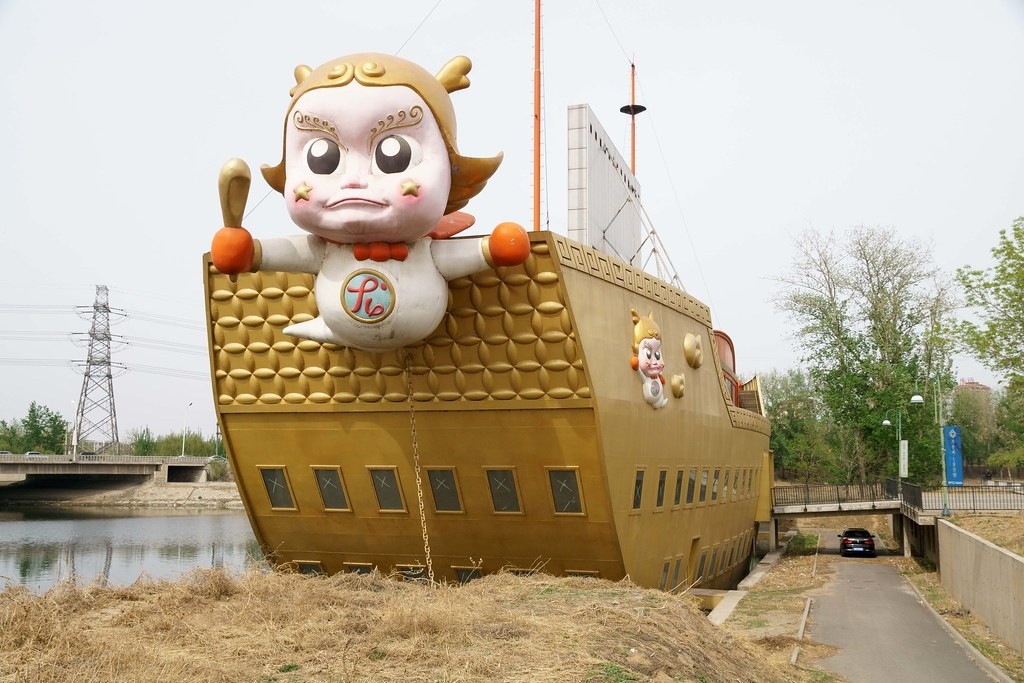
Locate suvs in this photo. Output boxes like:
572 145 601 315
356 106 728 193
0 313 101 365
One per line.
79 452 97 455
837 527 876 558
25 452 50 461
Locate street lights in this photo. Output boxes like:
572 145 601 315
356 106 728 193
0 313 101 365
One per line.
882 405 902 494
909 373 951 516
181 402 193 455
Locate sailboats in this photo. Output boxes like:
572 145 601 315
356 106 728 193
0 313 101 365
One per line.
204 0 772 591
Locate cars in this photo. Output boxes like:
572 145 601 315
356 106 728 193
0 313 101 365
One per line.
178 454 227 461
0 451 12 454
